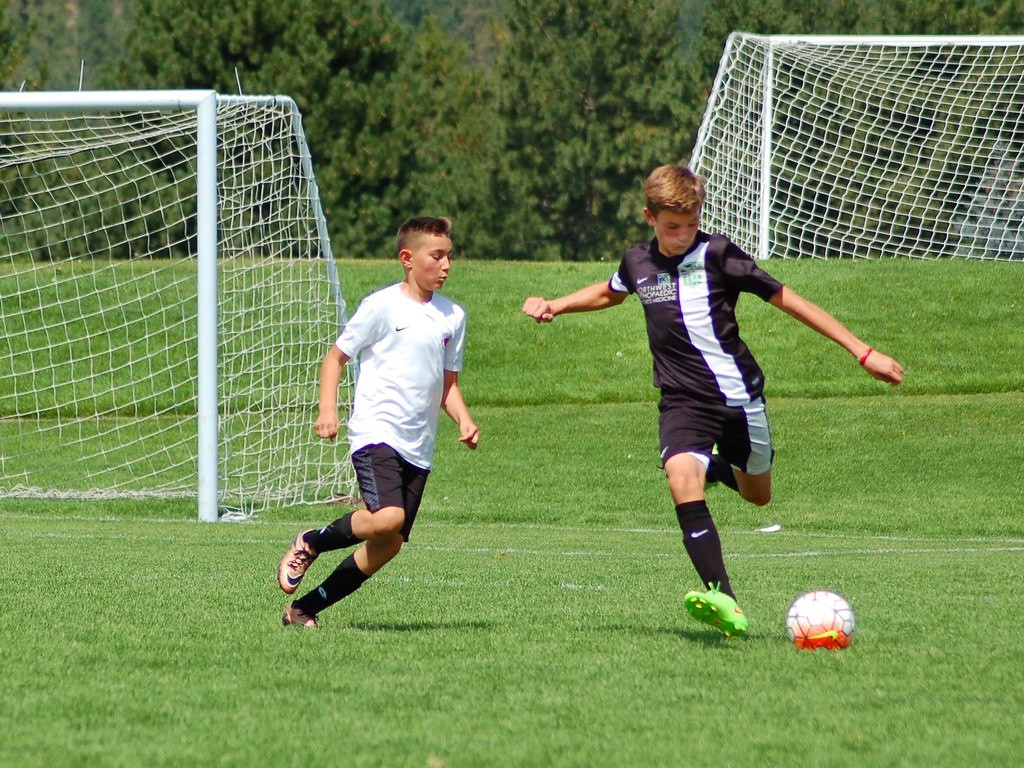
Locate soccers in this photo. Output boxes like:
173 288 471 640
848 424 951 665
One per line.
787 590 856 651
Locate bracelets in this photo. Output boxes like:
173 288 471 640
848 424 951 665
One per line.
859 347 873 365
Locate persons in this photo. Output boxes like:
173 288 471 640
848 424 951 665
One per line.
276 216 479 629
522 163 906 638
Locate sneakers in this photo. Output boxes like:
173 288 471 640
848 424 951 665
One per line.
683 581 748 637
704 443 719 492
282 600 319 630
277 529 320 595
